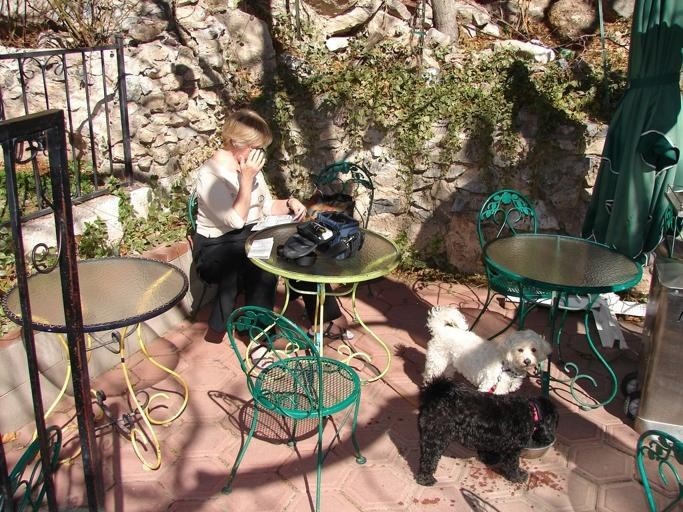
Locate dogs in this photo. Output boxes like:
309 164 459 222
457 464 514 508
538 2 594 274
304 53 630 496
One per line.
421 303 552 396
416 373 559 486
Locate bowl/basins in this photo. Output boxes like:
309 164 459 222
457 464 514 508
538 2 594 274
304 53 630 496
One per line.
520 432 556 459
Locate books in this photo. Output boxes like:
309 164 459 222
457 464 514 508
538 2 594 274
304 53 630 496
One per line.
246 236 274 259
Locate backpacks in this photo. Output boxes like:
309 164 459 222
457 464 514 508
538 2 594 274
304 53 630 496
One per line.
276 210 365 267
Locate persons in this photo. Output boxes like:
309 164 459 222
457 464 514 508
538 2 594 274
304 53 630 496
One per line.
195 109 353 368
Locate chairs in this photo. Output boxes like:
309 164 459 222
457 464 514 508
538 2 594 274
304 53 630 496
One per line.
636 430 683 512
188 185 198 231
468 188 567 339
317 163 376 298
221 305 366 512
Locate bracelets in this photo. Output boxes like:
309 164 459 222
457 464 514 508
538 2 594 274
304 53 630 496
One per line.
286 197 292 210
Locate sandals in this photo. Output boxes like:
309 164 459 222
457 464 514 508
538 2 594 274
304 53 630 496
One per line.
308 321 354 341
247 333 275 370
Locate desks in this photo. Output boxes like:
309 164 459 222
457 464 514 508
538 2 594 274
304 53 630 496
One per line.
480 233 644 410
244 221 402 384
2 256 189 469
